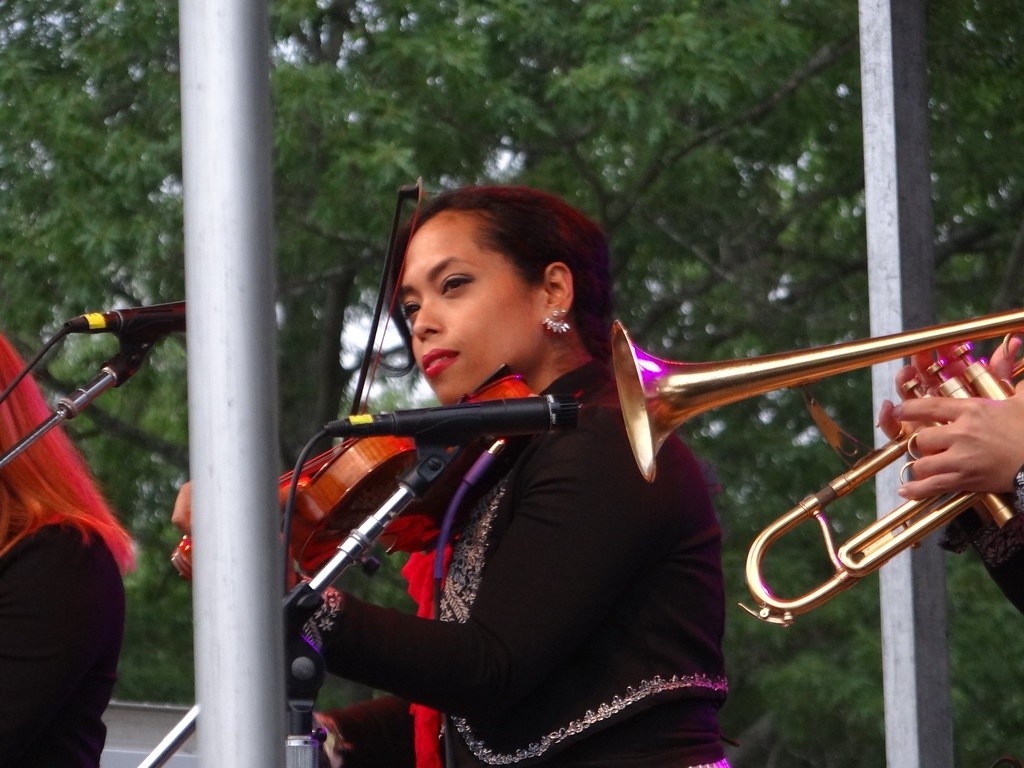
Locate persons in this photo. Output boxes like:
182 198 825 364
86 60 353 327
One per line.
0 332 133 768
172 183 730 768
879 336 1024 614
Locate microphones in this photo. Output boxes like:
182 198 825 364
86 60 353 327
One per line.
324 394 578 437
63 300 186 333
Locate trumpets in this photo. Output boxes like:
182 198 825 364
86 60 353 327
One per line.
604 306 1024 627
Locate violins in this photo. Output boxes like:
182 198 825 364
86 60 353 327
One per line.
172 373 545 588
